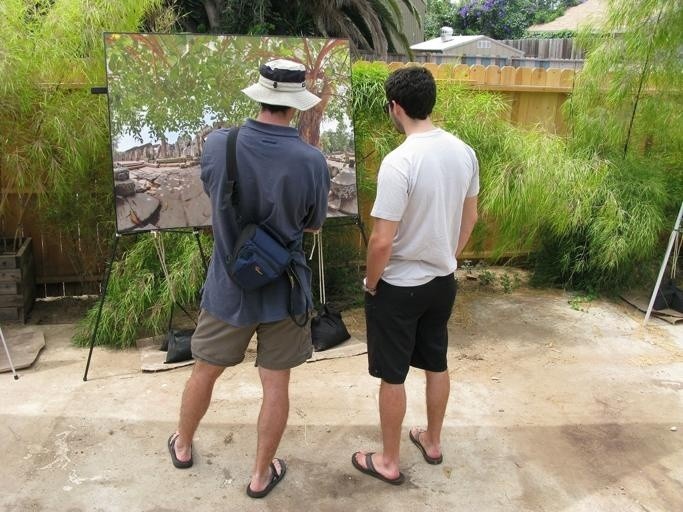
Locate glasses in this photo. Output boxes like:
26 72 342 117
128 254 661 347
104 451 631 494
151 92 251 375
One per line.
383 102 393 114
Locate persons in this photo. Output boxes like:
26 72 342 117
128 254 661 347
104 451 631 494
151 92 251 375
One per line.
350 65 481 487
167 58 330 497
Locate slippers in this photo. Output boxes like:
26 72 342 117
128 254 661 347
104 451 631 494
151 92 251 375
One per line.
246 457 286 498
168 432 193 469
409 425 443 465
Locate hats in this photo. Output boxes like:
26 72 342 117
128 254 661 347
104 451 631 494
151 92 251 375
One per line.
240 59 322 112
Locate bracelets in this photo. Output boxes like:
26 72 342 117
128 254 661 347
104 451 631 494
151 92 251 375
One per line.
360 276 377 294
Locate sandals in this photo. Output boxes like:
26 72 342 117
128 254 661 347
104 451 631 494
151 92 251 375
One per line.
352 450 405 485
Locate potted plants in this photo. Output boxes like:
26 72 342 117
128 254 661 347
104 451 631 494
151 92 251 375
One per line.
0 1 158 327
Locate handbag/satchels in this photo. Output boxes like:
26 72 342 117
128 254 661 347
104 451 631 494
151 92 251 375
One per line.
310 303 351 352
163 328 196 364
225 221 293 291
